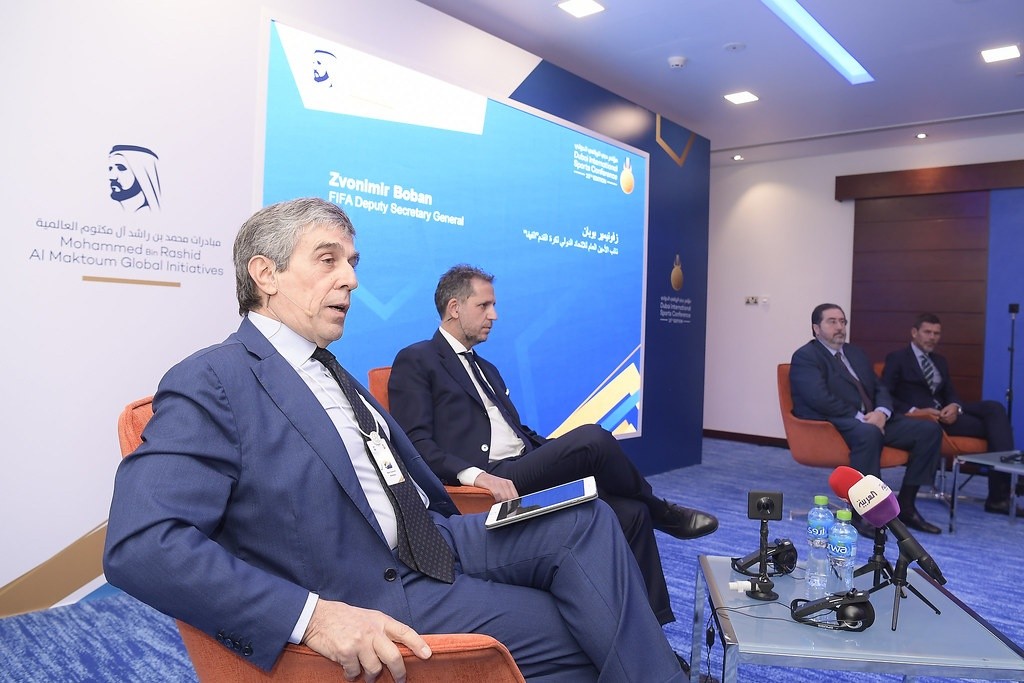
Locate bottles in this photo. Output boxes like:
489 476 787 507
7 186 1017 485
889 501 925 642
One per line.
806 495 859 608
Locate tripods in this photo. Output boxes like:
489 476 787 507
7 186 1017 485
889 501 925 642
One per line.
853 525 941 631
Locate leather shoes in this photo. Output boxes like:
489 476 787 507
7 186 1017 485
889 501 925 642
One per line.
984 498 1024 518
653 499 718 540
675 653 691 671
852 512 888 542
898 508 943 534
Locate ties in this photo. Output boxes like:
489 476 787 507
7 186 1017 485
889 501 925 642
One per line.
921 355 941 408
458 350 543 455
835 351 873 414
311 347 455 584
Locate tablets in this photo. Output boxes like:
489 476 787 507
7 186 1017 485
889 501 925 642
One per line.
484 476 599 530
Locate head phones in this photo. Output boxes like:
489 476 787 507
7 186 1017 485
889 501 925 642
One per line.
790 598 876 631
730 547 797 578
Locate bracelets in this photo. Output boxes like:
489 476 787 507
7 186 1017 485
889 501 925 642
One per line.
956 406 963 415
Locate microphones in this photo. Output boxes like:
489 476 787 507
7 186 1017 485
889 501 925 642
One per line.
277 290 314 318
458 317 470 340
848 474 948 585
828 466 864 504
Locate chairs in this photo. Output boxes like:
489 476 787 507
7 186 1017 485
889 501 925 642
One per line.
874 364 989 520
118 394 526 682
777 363 909 521
368 365 499 516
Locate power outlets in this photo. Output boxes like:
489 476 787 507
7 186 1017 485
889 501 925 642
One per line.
744 296 759 305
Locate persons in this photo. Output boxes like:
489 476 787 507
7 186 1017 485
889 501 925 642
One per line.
883 313 1024 517
104 200 691 683
389 265 719 670
788 303 942 541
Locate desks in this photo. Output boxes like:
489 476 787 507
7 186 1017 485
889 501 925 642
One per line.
688 554 1024 683
948 450 1024 534
